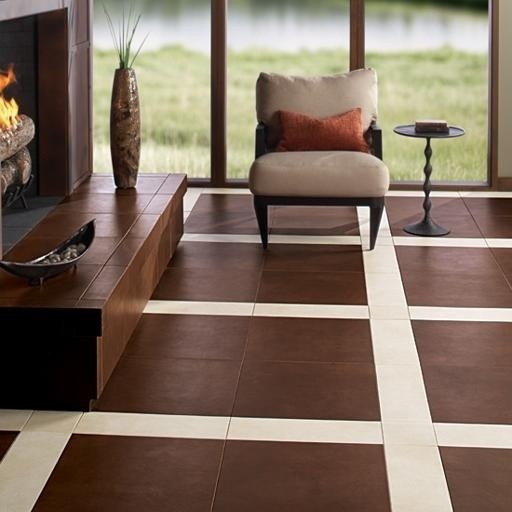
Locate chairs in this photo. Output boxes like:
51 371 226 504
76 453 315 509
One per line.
248 68 391 251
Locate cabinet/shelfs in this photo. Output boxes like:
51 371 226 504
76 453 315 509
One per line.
0 173 187 412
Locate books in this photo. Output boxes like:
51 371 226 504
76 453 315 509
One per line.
415 121 449 133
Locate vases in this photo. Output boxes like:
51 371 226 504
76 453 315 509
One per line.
109 67 141 188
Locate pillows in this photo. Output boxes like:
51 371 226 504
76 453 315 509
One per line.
272 108 369 153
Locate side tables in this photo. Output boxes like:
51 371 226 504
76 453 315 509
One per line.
393 124 465 236
